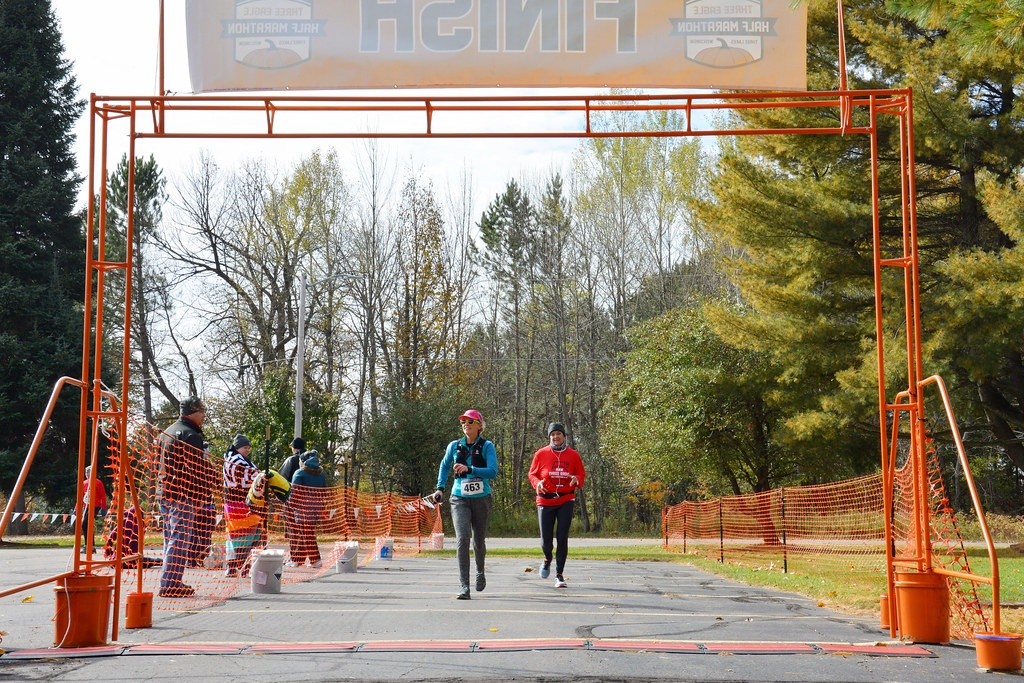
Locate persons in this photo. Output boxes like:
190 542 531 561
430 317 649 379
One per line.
152 394 211 598
185 502 215 567
432 409 499 599
79 466 107 555
528 423 585 588
275 437 326 568
222 434 270 578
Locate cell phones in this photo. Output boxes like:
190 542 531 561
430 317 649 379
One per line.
432 494 441 505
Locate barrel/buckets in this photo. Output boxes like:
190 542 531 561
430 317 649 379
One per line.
334 541 358 574
54 575 113 648
250 548 284 594
894 572 951 645
879 593 898 630
432 533 444 550
125 592 154 628
974 630 1024 671
375 537 393 561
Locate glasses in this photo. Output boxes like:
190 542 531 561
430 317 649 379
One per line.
460 420 480 425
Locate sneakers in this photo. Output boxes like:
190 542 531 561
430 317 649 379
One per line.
555 574 567 588
456 588 470 599
475 570 486 591
540 556 553 578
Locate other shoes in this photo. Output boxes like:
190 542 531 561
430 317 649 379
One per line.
181 584 192 589
91 548 96 553
285 560 299 566
310 561 322 567
159 588 195 598
80 546 86 553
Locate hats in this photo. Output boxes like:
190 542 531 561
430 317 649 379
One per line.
300 449 318 463
179 396 207 416
292 437 305 449
458 409 483 424
233 434 252 449
548 422 565 439
